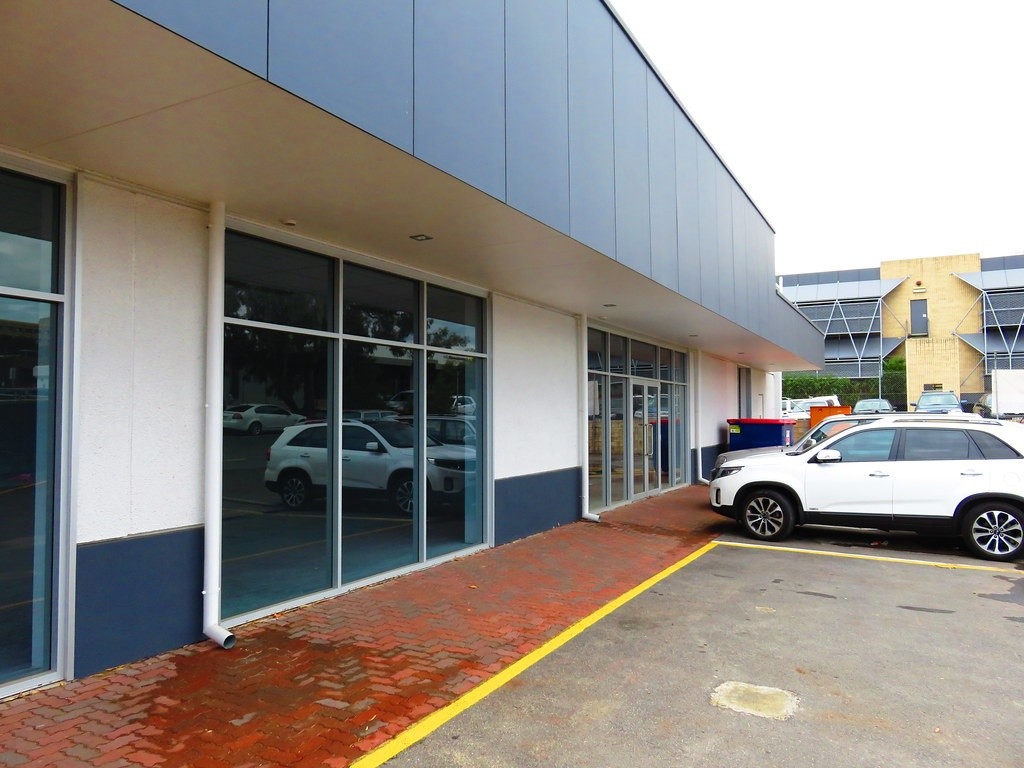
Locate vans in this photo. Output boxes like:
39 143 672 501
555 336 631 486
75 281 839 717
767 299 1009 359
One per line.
345 409 399 423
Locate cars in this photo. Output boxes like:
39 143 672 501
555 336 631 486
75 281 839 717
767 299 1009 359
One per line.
782 394 844 420
300 407 345 425
598 392 680 421
384 389 414 415
852 399 898 421
974 394 1014 421
433 395 477 417
223 403 306 435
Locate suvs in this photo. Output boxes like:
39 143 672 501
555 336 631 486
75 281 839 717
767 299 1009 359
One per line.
262 417 478 520
911 390 968 413
708 410 1024 563
382 416 477 449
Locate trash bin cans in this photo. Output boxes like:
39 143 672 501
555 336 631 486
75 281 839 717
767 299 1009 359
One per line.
648 418 681 473
724 418 797 452
